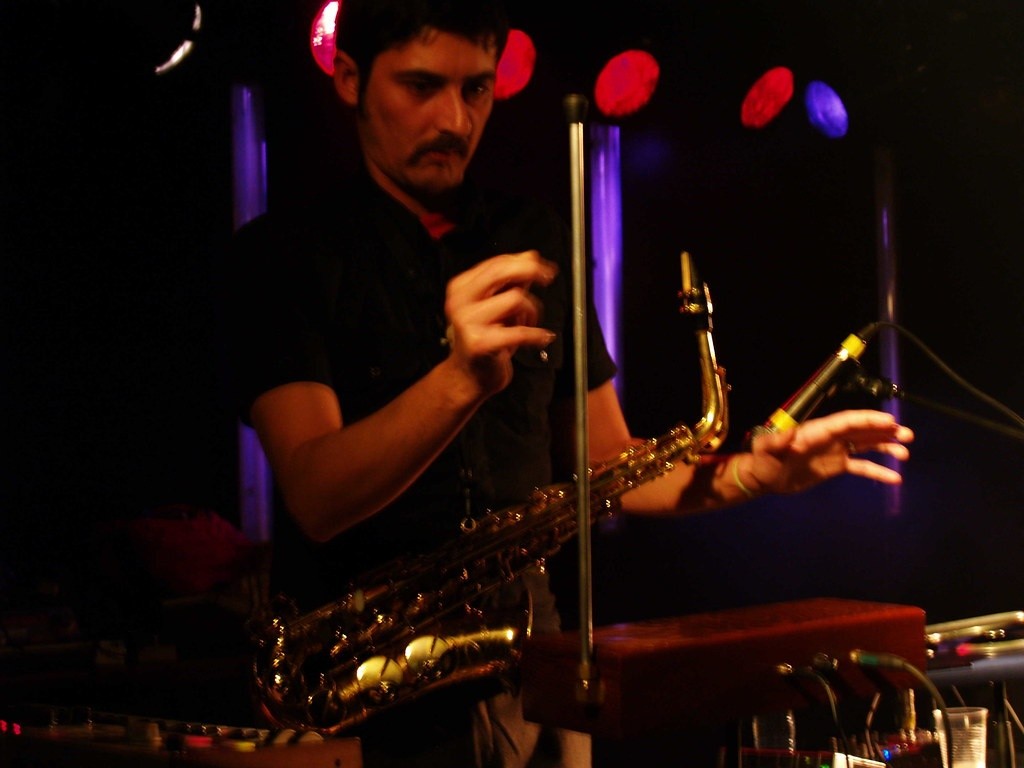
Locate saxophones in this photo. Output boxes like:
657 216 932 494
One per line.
240 248 731 739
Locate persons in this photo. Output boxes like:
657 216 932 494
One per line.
217 1 915 768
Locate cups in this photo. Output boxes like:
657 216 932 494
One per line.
934 708 989 768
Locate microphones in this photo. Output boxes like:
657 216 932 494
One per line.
764 323 880 447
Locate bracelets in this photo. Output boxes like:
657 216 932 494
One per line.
730 452 758 502
